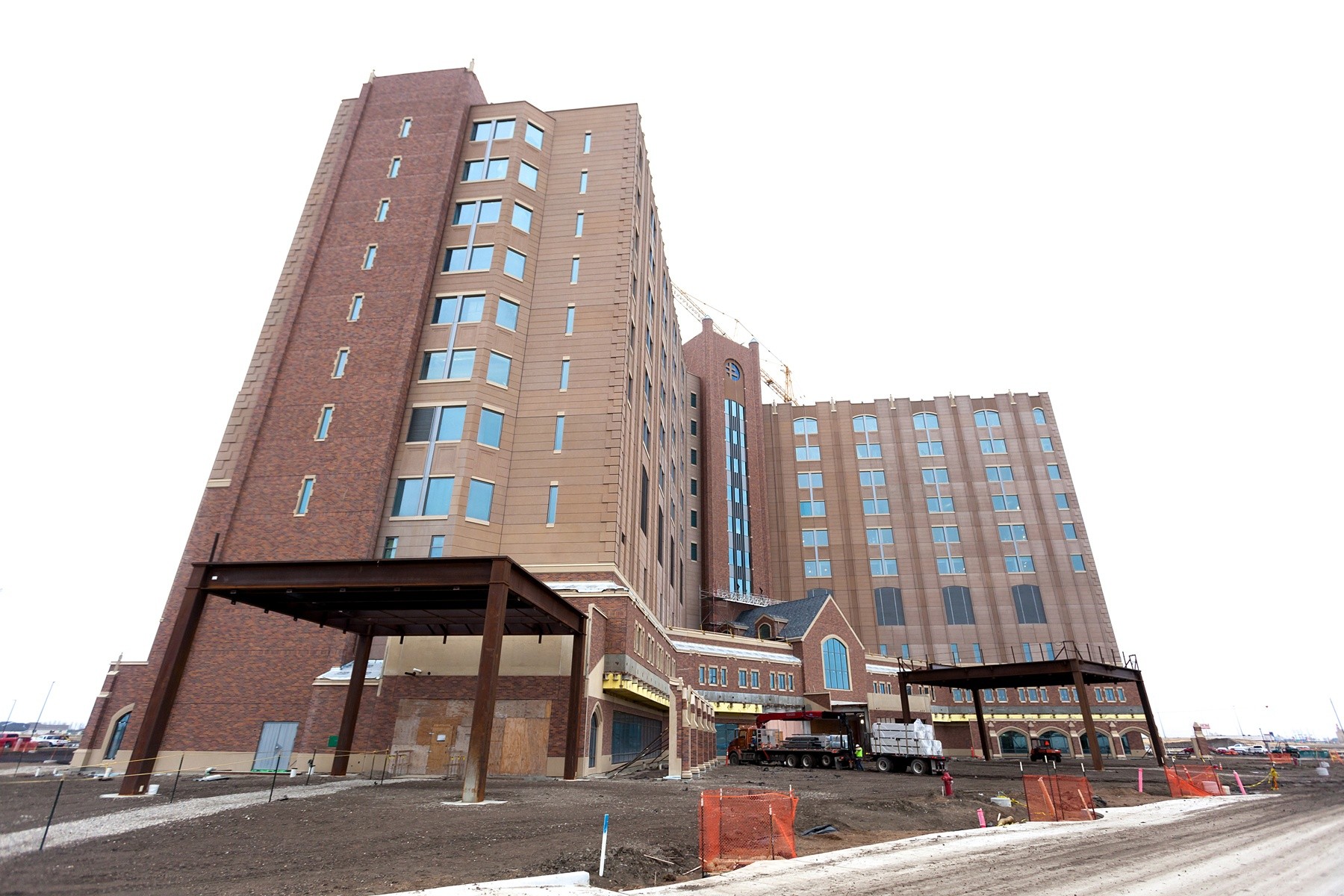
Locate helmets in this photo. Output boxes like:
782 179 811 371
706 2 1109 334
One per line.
856 744 859 747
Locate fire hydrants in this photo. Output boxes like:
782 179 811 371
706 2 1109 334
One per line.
940 770 953 797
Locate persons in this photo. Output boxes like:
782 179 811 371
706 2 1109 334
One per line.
855 744 865 771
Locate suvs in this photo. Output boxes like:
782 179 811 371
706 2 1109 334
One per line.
36 733 68 747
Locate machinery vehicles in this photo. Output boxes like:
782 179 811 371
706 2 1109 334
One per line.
727 704 857 771
1031 736 1063 763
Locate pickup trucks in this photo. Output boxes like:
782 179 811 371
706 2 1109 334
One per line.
1184 743 1310 755
0 732 32 749
